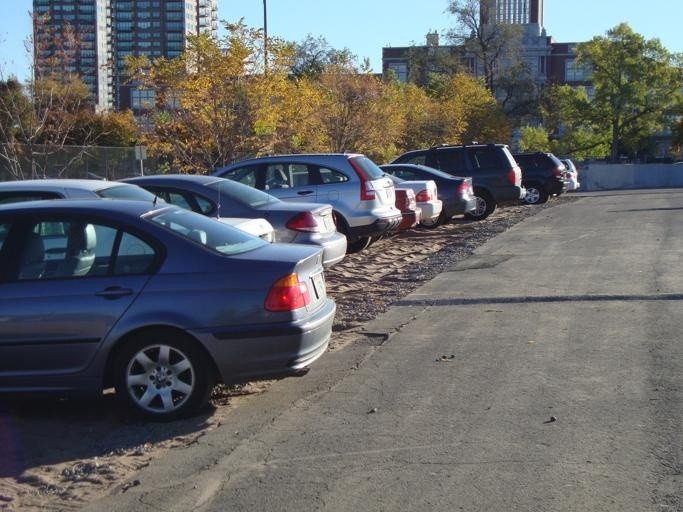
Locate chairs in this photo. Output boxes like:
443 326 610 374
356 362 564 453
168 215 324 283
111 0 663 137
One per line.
272 166 292 187
17 224 96 278
